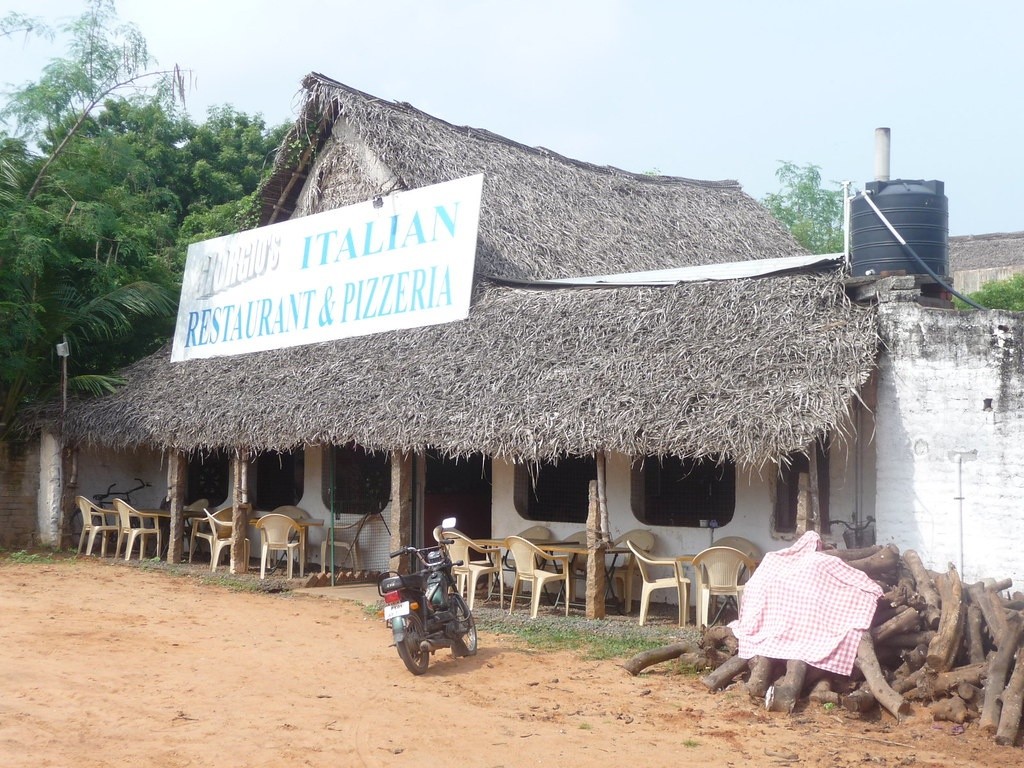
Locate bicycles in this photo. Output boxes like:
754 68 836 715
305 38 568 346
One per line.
69 478 151 551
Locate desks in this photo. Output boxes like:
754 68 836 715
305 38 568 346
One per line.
536 544 649 616
138 509 215 561
676 554 763 628
248 518 324 575
472 538 580 609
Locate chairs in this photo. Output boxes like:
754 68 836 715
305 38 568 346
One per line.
182 499 209 554
321 514 372 574
113 498 162 561
189 503 251 564
255 505 311 580
204 508 251 574
433 525 763 629
75 495 121 557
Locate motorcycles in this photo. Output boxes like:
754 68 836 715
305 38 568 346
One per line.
372 517 477 675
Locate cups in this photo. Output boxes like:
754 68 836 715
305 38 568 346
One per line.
699 520 708 527
